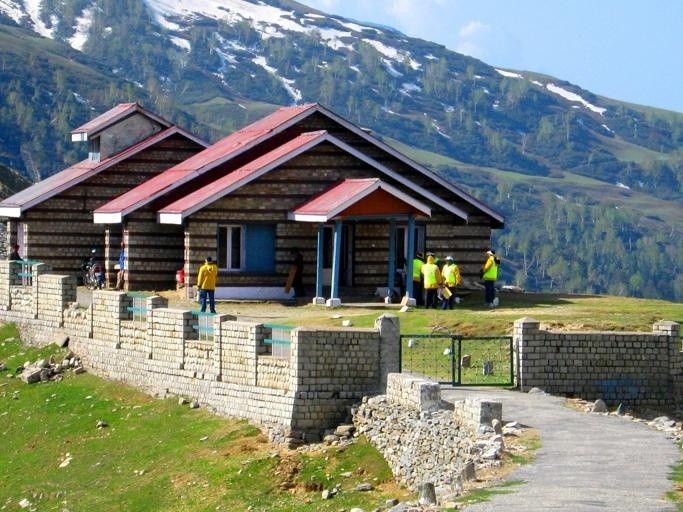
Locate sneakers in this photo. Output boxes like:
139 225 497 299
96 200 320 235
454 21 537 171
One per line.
456 297 460 303
483 297 499 307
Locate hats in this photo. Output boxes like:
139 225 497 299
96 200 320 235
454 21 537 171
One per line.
426 251 435 256
444 255 454 262
417 252 424 260
486 250 495 255
205 257 211 262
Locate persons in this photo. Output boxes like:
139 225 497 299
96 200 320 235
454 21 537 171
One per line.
197 256 218 313
412 251 462 310
111 241 125 291
7 243 23 260
480 250 500 307
289 245 306 298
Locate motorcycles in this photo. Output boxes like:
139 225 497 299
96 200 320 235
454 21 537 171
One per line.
80 249 102 290
